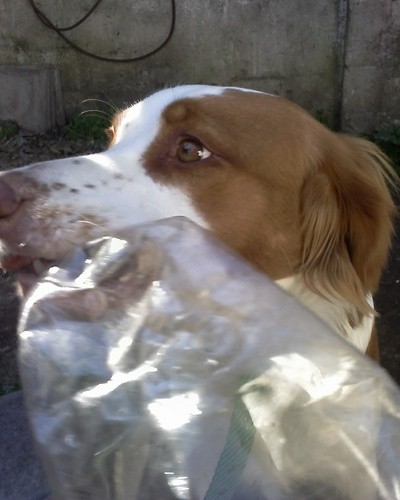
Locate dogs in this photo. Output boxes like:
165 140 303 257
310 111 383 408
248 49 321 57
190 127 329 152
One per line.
0 82 400 500
